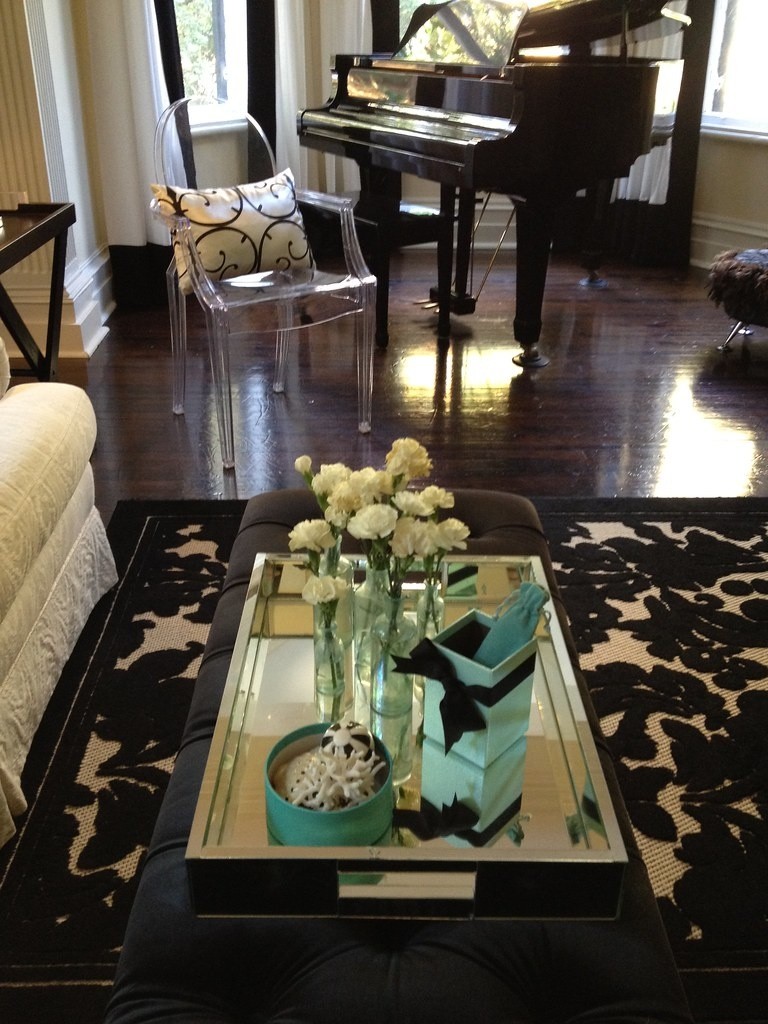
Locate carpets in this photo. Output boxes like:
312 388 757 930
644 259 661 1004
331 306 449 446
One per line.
0 497 768 1024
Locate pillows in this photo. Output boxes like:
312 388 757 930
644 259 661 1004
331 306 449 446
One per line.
150 168 318 295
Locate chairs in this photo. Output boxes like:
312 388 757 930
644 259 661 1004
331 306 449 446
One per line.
151 94 378 470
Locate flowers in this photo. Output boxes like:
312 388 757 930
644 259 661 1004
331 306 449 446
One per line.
283 436 470 605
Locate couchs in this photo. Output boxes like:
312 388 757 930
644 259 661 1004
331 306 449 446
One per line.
703 247 768 353
0 383 121 849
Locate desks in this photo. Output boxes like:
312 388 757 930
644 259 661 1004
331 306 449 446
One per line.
183 552 630 922
0 201 76 385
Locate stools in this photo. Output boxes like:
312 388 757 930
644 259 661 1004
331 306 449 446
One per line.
296 189 451 348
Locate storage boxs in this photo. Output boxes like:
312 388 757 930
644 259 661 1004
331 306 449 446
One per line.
262 722 395 848
388 609 539 769
395 735 528 856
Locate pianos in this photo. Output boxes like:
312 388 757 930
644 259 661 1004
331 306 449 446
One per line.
297 53 660 360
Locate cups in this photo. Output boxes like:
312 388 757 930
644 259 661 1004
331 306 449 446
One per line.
263 721 400 890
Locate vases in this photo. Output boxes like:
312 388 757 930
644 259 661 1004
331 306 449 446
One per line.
304 558 443 787
